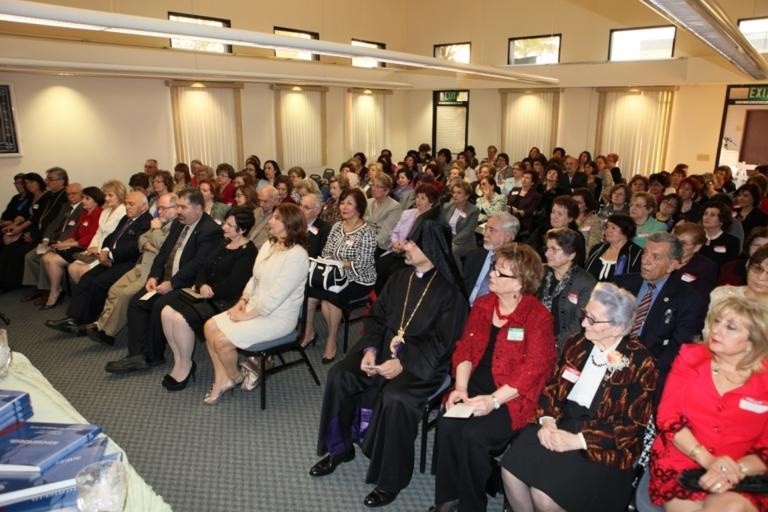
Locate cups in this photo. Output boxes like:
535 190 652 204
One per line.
74 458 128 512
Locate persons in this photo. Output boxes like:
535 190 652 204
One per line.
1 142 768 510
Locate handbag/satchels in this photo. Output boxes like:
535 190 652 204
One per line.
238 355 264 393
305 255 349 294
678 464 767 495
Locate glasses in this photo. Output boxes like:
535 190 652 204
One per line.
232 192 247 199
628 202 657 208
489 264 525 282
578 305 617 327
369 183 388 189
44 176 66 185
155 202 178 212
222 220 240 229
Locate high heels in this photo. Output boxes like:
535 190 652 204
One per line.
161 359 197 392
298 331 338 365
202 373 244 405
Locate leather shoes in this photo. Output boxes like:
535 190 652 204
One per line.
425 495 462 512
44 315 117 346
16 284 66 311
105 352 163 376
307 443 357 478
363 474 413 508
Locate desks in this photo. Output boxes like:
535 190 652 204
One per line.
0 350 174 512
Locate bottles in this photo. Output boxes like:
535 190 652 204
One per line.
0 328 11 378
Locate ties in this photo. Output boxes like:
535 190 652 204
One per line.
162 225 190 282
60 206 74 234
627 280 660 345
117 217 134 244
